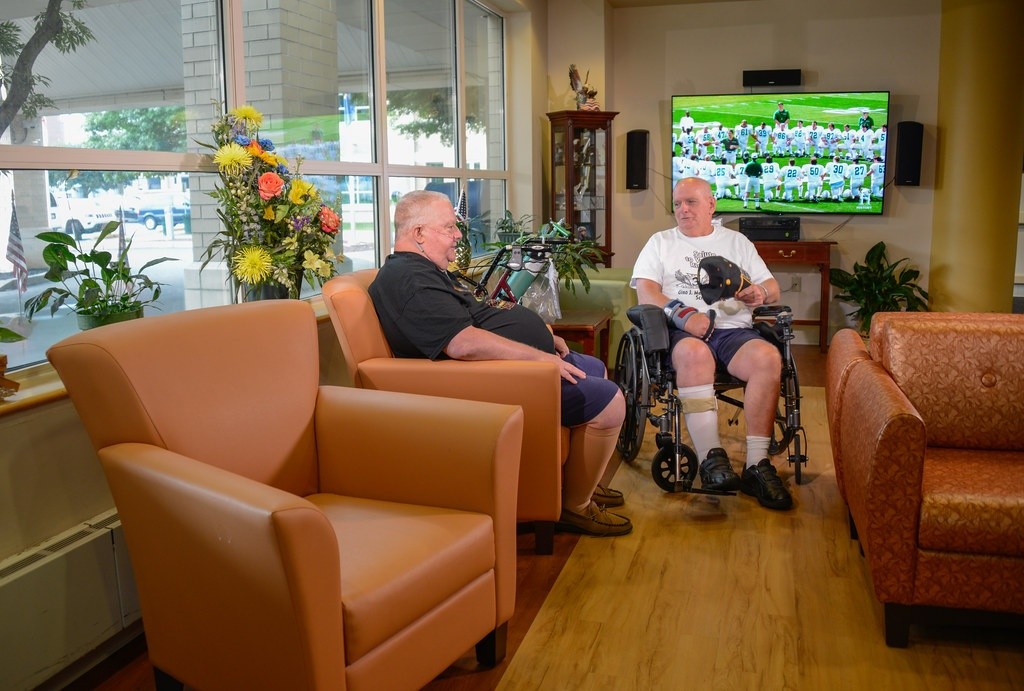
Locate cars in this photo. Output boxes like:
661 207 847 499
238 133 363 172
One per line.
49 188 116 241
115 201 140 222
139 196 191 231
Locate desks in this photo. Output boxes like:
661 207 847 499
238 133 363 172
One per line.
754 239 838 353
553 308 613 371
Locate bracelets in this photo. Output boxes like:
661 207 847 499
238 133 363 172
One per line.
753 283 769 303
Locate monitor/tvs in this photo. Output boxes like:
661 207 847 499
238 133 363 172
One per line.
671 91 890 216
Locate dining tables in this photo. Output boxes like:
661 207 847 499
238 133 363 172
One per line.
791 277 801 292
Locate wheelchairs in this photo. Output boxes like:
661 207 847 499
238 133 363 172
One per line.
612 303 809 498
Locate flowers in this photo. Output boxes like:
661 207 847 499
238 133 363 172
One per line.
195 100 342 301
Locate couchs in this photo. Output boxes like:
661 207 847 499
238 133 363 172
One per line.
320 268 569 556
826 311 1024 645
47 299 522 691
555 267 637 368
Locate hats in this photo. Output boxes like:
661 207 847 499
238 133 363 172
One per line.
752 153 758 158
862 109 868 113
697 256 752 305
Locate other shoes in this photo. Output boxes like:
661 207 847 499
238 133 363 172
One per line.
756 207 762 210
560 484 633 536
743 206 747 208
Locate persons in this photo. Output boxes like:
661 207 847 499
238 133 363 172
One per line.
368 191 633 535
669 102 887 211
629 177 794 511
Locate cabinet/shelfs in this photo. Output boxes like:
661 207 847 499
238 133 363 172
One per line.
547 109 620 266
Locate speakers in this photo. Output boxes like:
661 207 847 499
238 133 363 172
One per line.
895 121 923 186
742 69 802 86
627 129 650 189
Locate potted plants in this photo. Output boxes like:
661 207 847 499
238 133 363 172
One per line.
492 210 537 242
25 220 179 330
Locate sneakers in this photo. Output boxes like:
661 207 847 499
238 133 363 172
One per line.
740 457 792 510
699 447 741 492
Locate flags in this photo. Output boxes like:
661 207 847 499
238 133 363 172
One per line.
118 209 132 279
6 190 28 293
455 191 466 227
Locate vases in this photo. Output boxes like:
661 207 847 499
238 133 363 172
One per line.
248 268 308 301
